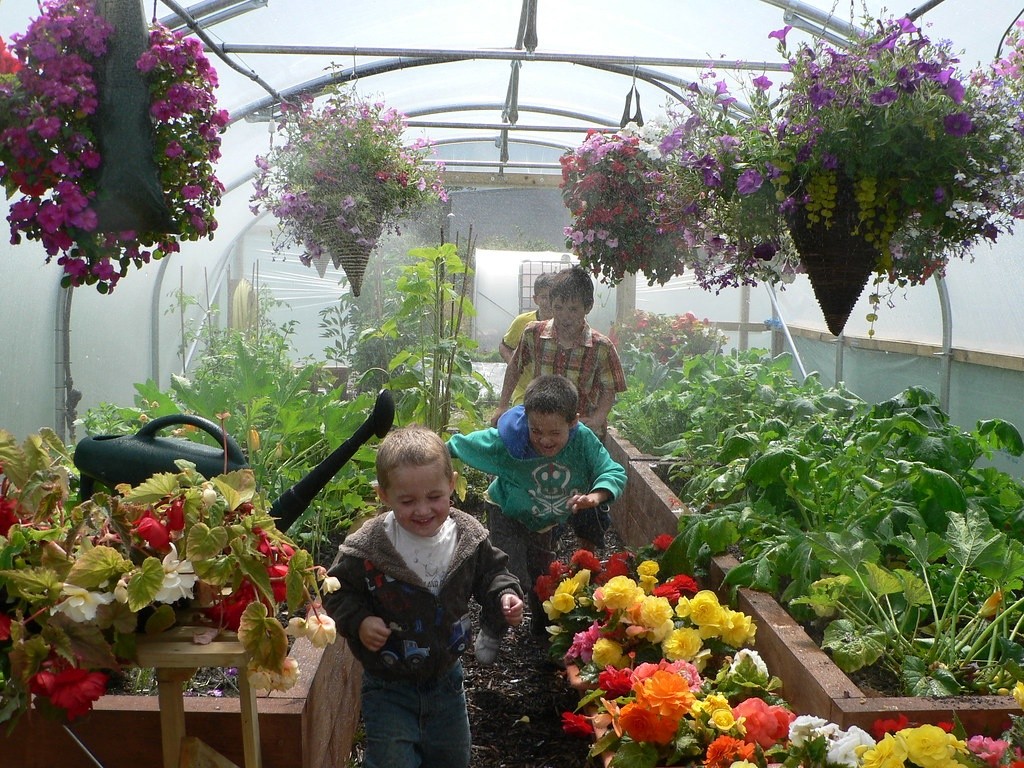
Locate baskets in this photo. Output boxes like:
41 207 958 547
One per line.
786 168 906 336
312 200 384 297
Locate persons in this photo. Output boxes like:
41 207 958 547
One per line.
317 425 524 768
441 374 630 666
488 267 628 567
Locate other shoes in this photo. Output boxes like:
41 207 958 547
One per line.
473 628 501 666
